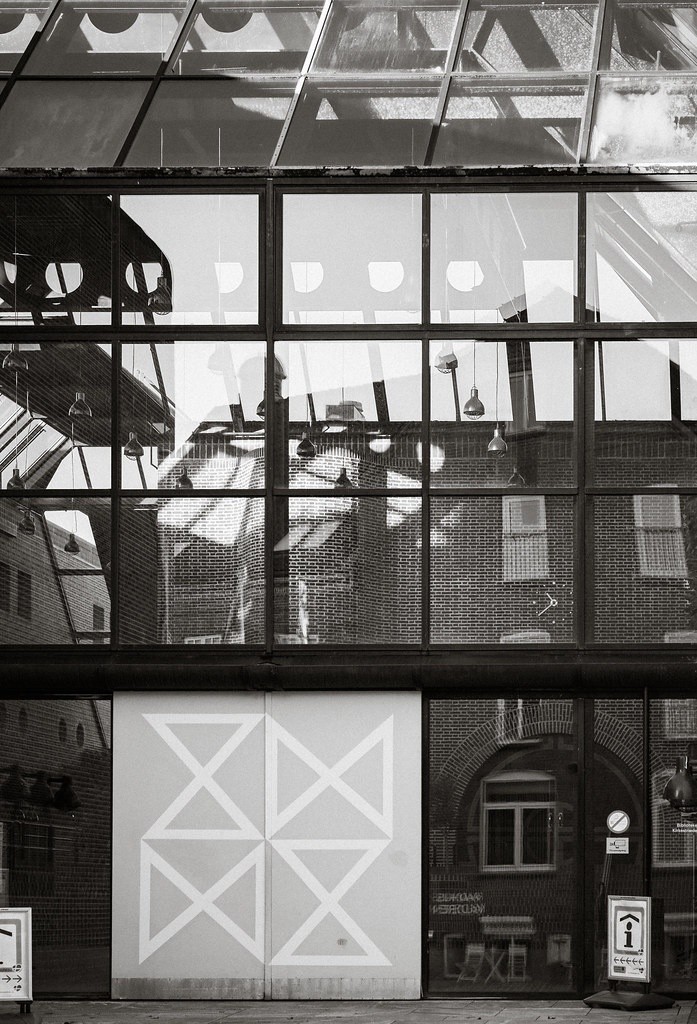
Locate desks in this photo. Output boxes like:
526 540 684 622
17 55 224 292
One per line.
464 933 532 983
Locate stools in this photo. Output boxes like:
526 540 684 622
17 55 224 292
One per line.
507 944 526 983
456 943 486 981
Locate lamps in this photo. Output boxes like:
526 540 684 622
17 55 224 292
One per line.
1 195 526 557
0 764 82 813
663 756 696 813
